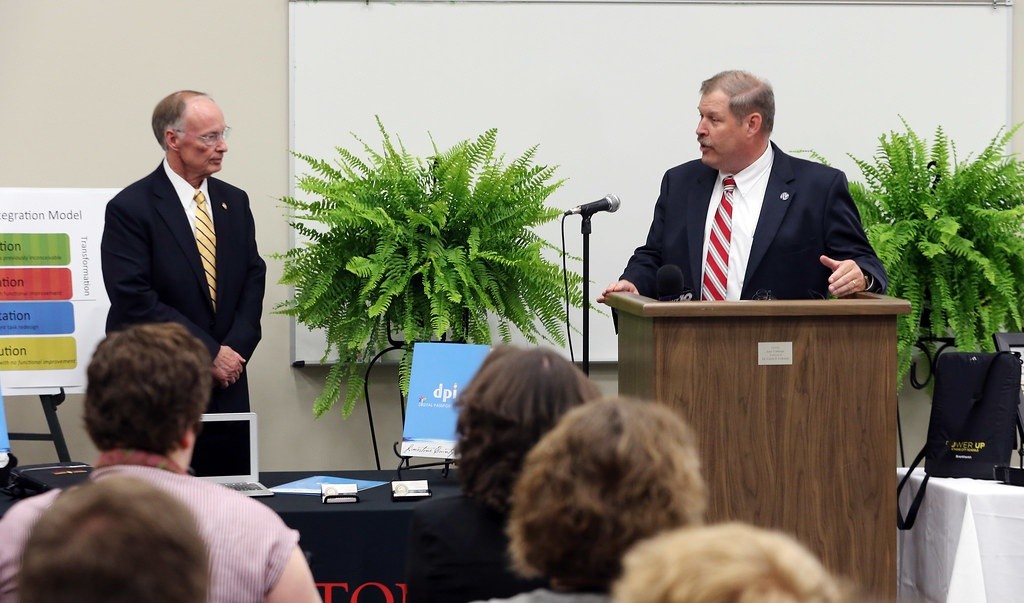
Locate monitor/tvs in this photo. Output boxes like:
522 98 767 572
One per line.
992 332 1024 443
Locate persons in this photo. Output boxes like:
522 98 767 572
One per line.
21 475 208 603
100 90 267 414
404 345 601 603
594 70 888 304
610 519 844 603
0 322 322 603
471 398 710 603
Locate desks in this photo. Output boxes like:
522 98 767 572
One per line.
0 468 488 603
896 466 1024 602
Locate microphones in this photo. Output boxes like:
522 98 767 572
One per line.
657 265 684 302
564 194 621 216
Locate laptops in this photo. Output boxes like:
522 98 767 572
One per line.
186 412 274 497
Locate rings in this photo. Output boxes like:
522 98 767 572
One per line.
852 280 856 288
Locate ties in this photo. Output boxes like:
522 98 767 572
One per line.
194 189 216 313
702 175 736 301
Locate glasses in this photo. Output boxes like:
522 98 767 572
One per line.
174 126 233 147
